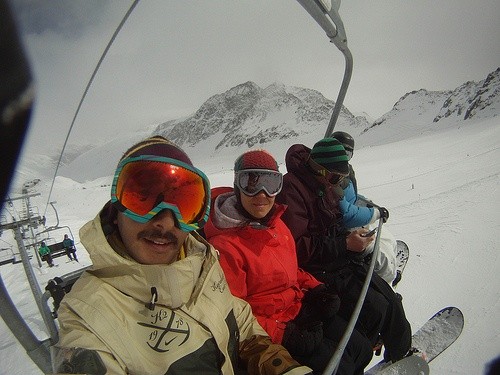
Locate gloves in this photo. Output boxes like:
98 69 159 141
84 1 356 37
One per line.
379 206 389 223
281 284 340 357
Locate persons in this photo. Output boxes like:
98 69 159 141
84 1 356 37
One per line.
55 139 314 375
39 242 54 266
63 234 78 262
204 151 373 375
275 138 464 375
329 132 409 287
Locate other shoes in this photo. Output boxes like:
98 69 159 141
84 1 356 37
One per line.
392 271 401 287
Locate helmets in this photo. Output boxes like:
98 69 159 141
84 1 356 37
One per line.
331 131 354 155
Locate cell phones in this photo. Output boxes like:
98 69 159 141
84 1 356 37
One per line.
365 227 378 237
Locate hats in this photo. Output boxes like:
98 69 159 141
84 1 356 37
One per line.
235 151 279 172
309 137 350 175
119 135 193 167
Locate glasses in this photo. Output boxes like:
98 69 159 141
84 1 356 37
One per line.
111 156 211 232
234 169 283 197
310 160 349 184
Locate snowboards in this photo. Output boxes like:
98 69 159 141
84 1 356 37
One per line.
395 239 409 292
374 356 430 375
361 307 464 374
